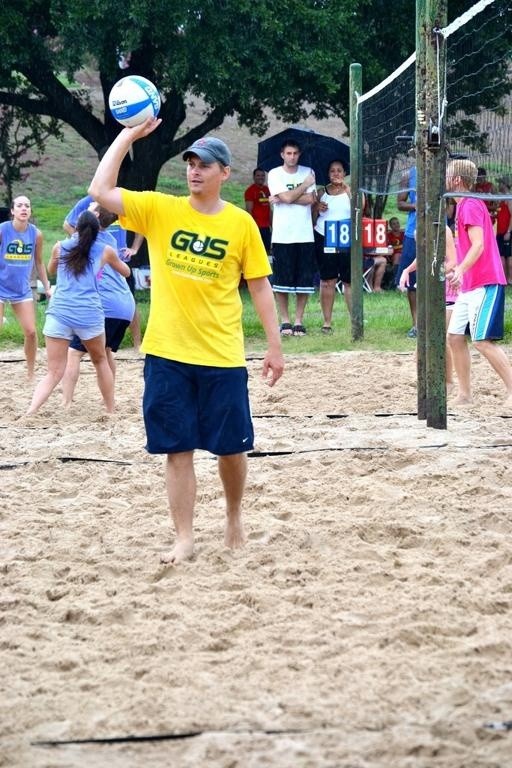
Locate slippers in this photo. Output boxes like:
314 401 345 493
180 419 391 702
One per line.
280 323 292 335
294 322 306 337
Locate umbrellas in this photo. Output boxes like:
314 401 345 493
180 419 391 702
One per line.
258 127 351 185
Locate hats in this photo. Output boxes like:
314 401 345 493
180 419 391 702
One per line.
183 136 232 166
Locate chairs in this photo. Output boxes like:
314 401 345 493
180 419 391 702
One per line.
335 259 373 295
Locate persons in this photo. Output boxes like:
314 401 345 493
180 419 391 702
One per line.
268 141 318 338
311 160 366 334
362 255 388 292
87 115 285 568
25 210 131 419
395 158 512 418
63 196 145 353
0 194 52 380
245 169 271 254
61 201 136 409
388 217 405 289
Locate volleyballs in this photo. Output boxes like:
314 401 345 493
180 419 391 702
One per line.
109 75 161 127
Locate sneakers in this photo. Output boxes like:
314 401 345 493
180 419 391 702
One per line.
410 326 416 337
320 323 332 333
